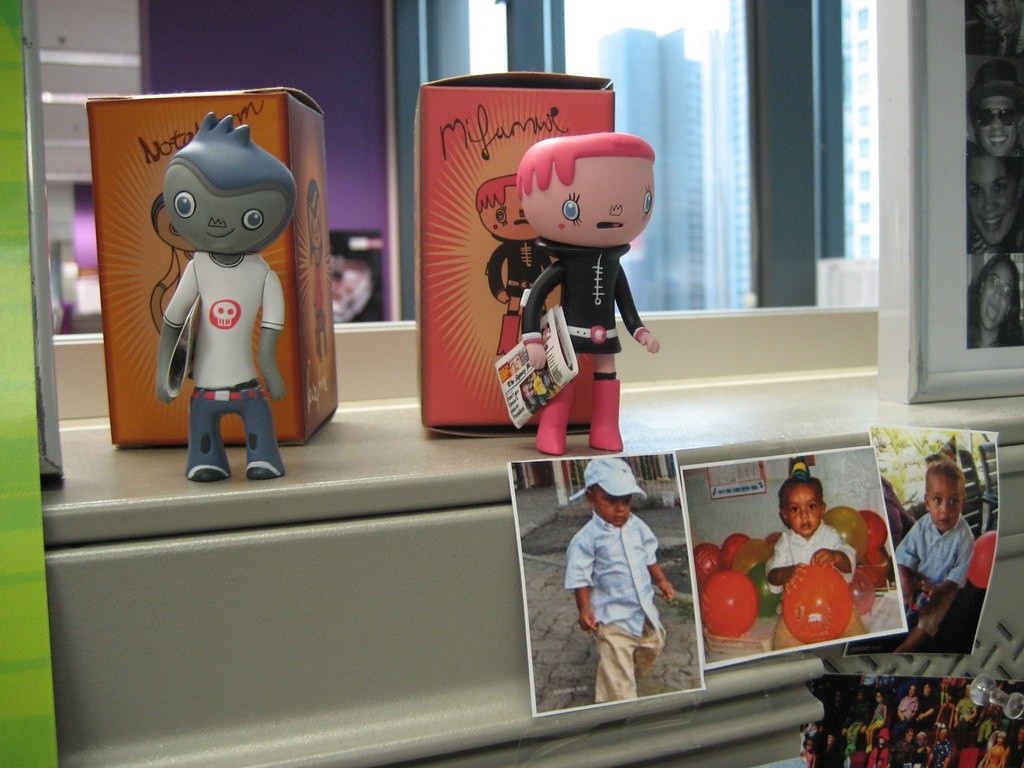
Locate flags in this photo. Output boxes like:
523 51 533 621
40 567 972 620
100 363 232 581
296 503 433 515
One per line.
938 436 958 461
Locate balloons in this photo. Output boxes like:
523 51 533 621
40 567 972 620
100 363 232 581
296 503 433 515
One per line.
968 532 998 588
694 506 889 644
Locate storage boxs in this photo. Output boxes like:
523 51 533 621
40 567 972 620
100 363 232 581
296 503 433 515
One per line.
87 87 337 445
415 72 615 437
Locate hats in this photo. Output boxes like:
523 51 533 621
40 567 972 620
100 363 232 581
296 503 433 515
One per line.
569 458 648 502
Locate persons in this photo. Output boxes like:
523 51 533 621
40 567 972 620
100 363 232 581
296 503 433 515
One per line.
800 679 1023 767
767 457 865 651
563 456 674 706
894 459 974 655
967 0 1024 347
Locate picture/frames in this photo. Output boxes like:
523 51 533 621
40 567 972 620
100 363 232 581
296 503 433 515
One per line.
876 0 1024 404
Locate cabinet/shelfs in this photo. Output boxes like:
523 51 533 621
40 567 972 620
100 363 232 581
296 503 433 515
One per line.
23 0 143 315
